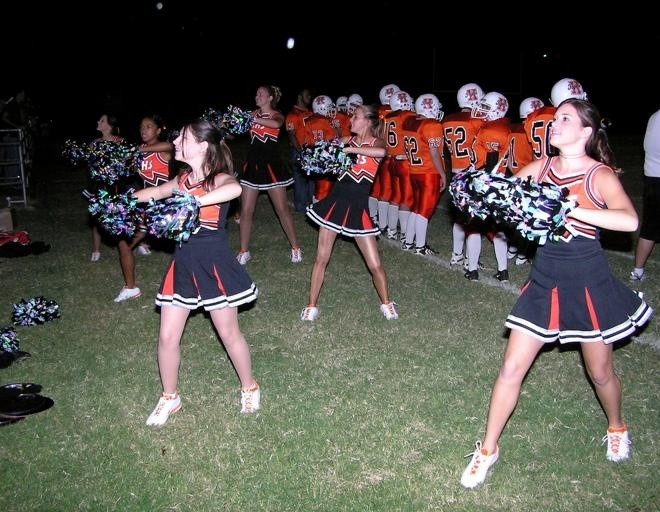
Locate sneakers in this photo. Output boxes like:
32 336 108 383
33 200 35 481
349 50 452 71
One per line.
291 247 302 262
239 382 260 414
465 270 478 281
380 303 398 321
413 244 439 256
462 443 499 487
606 424 629 463
236 251 250 265
114 286 141 304
464 260 488 271
302 307 319 321
494 269 508 283
146 392 182 426
138 246 151 255
402 241 417 251
400 234 407 243
507 250 518 259
630 272 648 282
449 252 467 266
90 252 100 262
388 231 400 240
515 256 533 266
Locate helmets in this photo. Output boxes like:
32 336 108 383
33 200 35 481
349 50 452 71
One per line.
347 93 363 113
379 84 400 105
520 98 544 118
458 84 485 110
551 79 586 107
472 92 508 121
389 91 414 111
336 97 348 111
415 94 444 121
312 96 337 118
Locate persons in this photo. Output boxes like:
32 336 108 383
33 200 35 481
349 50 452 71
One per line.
381 91 417 244
507 97 561 266
85 121 261 427
441 83 487 272
341 93 364 138
464 92 513 282
59 114 127 263
131 116 176 256
284 90 316 216
331 96 351 136
304 96 338 203
297 105 399 322
401 93 447 256
201 87 302 265
367 84 401 236
526 77 591 161
628 109 660 284
450 98 653 488
